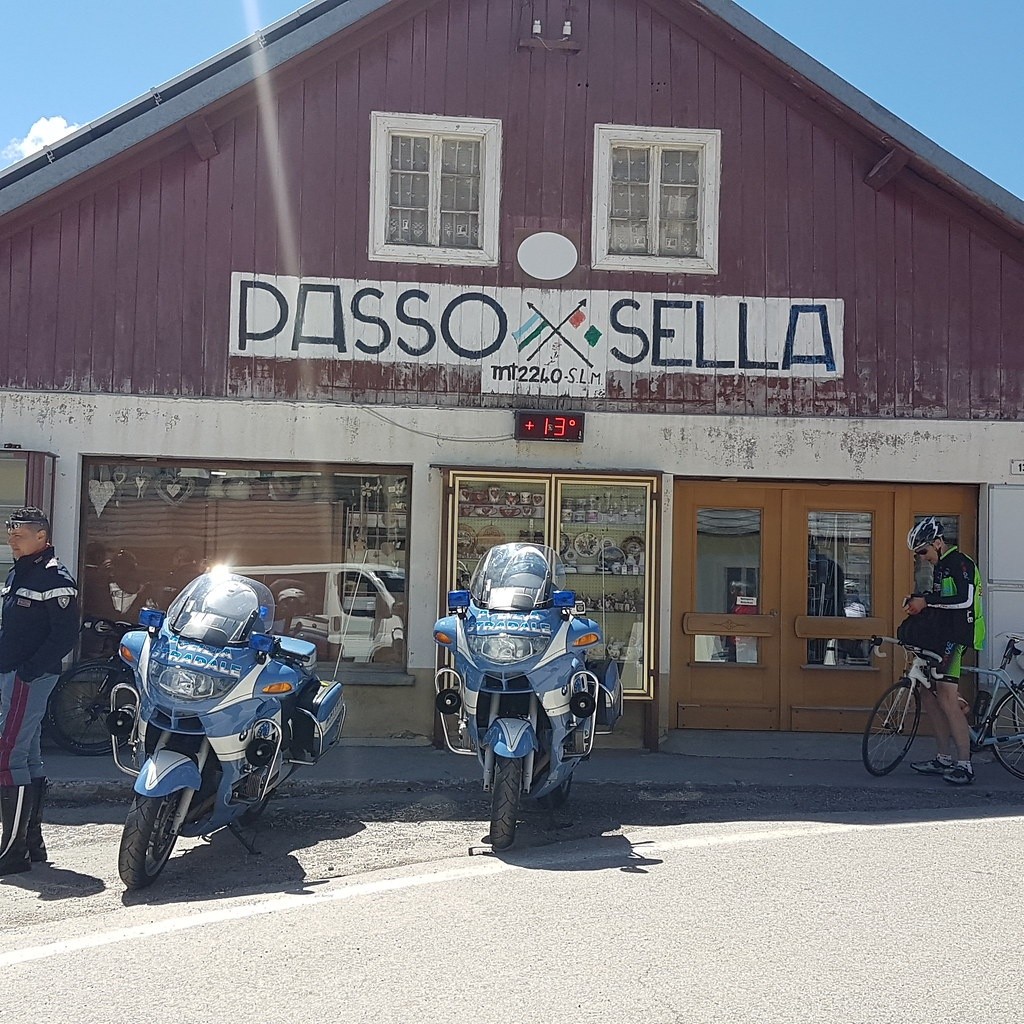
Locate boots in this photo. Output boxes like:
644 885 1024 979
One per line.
0 775 48 875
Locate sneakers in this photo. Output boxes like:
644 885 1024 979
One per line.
943 765 977 786
910 759 954 775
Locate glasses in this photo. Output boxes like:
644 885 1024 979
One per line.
5 519 43 529
914 548 927 561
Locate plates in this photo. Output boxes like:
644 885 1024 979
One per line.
477 526 506 557
620 536 644 565
600 536 616 550
574 531 599 558
598 547 627 566
564 548 578 561
560 530 570 555
458 523 479 555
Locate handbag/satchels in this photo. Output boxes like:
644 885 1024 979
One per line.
898 589 955 654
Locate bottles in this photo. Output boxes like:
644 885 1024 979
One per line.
589 493 644 524
638 551 644 575
627 553 636 575
201 474 335 502
557 561 625 574
534 528 544 545
972 691 992 716
956 693 970 714
528 518 536 542
519 529 530 543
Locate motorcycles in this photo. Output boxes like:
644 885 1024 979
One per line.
432 541 626 849
106 550 368 891
279 589 331 665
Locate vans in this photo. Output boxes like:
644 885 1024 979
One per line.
217 564 407 665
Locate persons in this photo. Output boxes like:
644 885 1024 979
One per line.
84 536 206 665
806 534 874 668
0 504 84 878
902 515 987 784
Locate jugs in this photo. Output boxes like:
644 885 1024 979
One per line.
608 644 619 659
620 644 629 661
609 637 625 656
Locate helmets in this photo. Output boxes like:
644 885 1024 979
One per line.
500 545 551 605
277 587 306 609
906 515 945 551
202 581 259 623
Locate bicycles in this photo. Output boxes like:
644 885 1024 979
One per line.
47 616 136 757
862 634 1023 779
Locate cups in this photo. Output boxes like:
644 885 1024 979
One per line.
574 511 585 524
562 508 574 522
459 484 545 519
562 497 575 510
574 498 587 512
586 510 603 524
